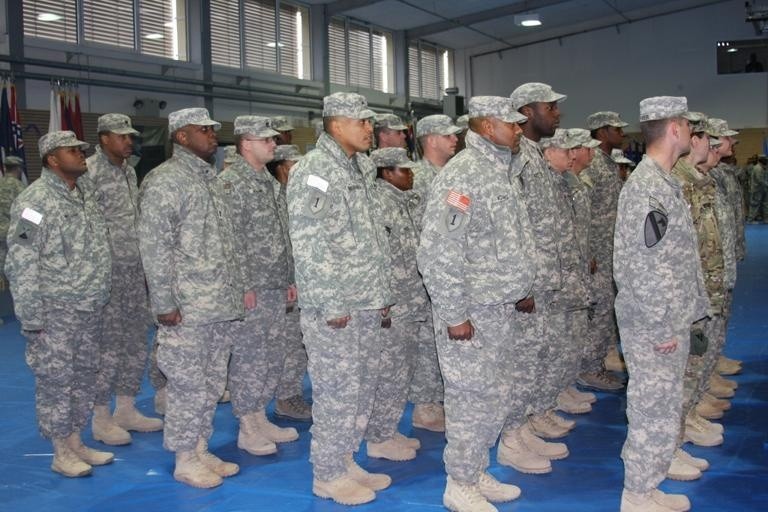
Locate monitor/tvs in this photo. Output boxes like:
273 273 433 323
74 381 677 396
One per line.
716 38 768 75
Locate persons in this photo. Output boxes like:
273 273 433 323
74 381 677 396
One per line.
136 82 765 510
1 114 166 477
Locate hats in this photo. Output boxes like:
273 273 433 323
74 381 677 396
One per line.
468 83 637 171
322 92 470 170
4 156 24 167
37 108 304 163
638 97 741 149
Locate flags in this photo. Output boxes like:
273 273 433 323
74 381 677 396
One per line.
1 81 30 184
49 87 83 142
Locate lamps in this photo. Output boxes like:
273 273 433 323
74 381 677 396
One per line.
513 13 542 28
728 44 740 55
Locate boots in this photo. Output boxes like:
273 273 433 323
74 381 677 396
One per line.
50 389 167 479
620 355 744 511
195 439 240 478
236 395 313 456
442 345 626 511
311 401 446 508
173 450 224 489
